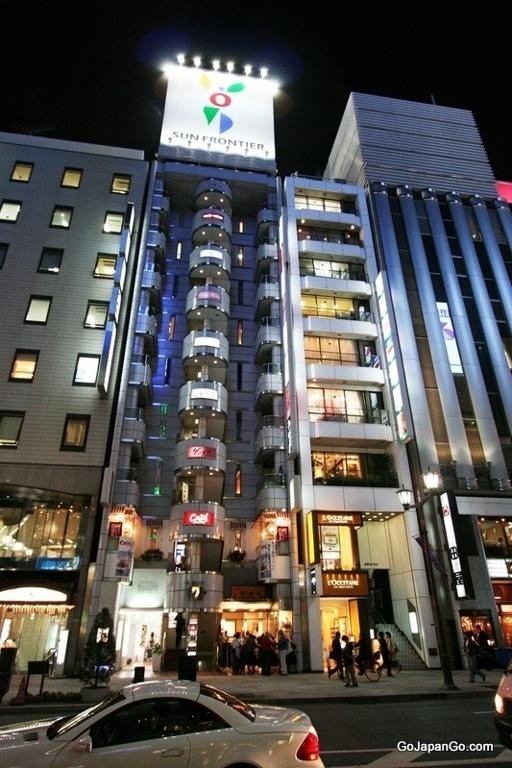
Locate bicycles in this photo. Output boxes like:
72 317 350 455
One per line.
336 653 383 682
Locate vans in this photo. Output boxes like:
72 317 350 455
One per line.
493 663 512 749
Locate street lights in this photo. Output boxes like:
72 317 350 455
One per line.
394 464 461 691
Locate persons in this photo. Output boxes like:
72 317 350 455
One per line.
474 624 493 671
174 612 186 648
231 629 289 676
328 631 400 687
462 631 486 682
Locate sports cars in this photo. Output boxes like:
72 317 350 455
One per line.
0 677 328 767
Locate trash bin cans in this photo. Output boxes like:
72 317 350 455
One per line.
178 655 196 682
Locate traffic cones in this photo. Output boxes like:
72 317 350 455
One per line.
14 675 26 705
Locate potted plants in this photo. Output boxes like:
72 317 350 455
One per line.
151 644 163 673
77 606 118 702
